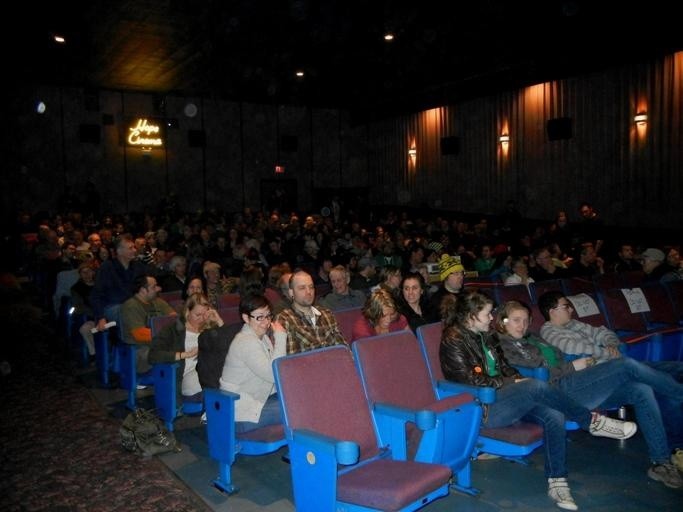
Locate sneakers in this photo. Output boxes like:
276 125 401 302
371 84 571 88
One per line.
589 412 637 440
670 449 683 473
647 463 682 488
548 477 578 511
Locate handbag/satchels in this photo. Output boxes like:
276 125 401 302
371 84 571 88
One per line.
119 406 176 458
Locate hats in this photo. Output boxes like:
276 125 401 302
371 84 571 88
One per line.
78 263 96 274
641 248 665 261
428 242 443 252
438 253 465 283
145 232 158 240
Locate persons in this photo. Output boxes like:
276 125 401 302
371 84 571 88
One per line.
435 290 636 511
529 289 681 374
489 299 680 489
144 294 225 426
1 195 682 375
217 294 288 465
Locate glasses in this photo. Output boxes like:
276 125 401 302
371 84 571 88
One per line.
553 303 574 311
249 314 273 321
536 254 553 259
